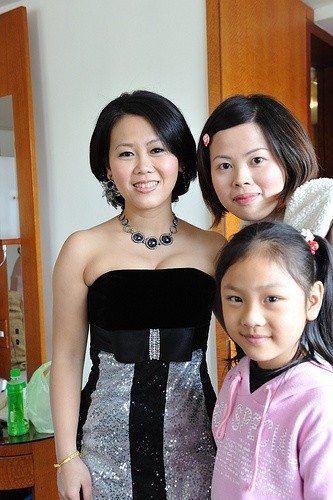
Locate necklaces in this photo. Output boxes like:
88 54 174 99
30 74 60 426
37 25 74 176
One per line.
119 211 178 250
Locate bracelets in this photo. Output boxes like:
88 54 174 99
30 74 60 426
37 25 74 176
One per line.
54 451 80 469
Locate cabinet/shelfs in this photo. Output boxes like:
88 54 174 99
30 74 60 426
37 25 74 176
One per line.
2 420 58 500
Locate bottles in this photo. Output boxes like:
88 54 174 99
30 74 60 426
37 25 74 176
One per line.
6 367 30 436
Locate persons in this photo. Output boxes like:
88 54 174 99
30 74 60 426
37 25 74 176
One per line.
50 90 232 500
211 222 333 500
196 94 333 249
